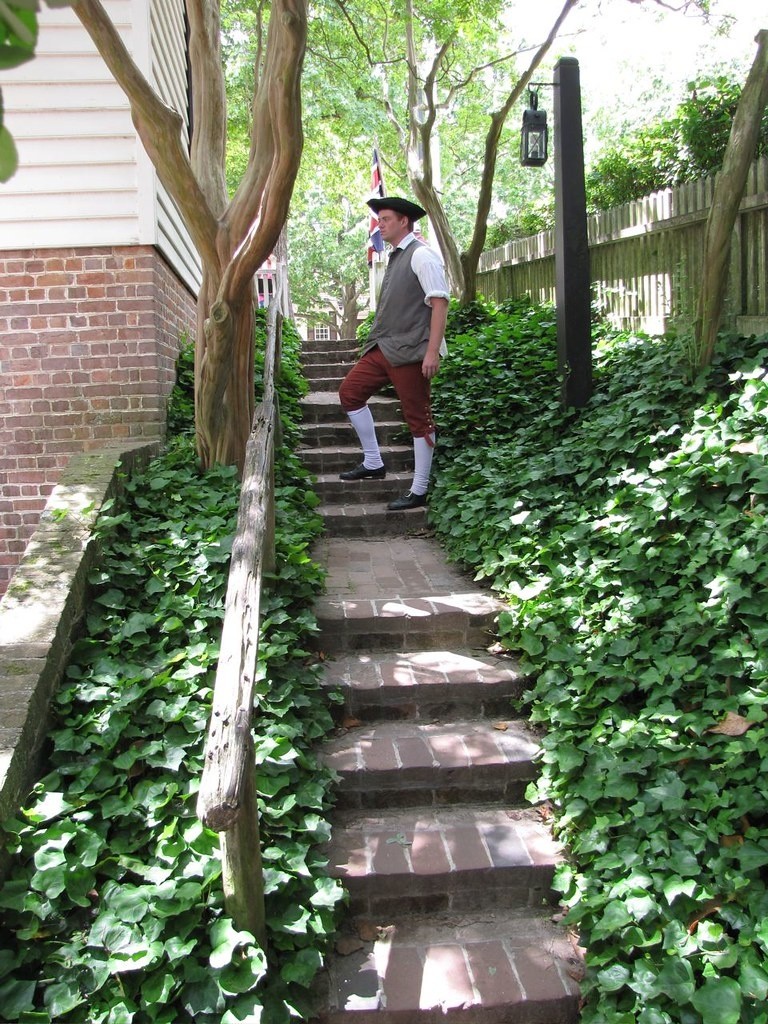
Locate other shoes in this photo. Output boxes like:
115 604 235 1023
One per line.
339 463 386 480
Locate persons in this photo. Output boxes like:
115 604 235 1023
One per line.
339 196 450 510
412 221 430 246
256 254 277 309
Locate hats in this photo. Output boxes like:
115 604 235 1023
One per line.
366 197 427 223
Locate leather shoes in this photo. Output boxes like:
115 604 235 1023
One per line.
387 490 428 510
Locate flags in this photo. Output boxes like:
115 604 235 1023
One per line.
367 136 388 268
